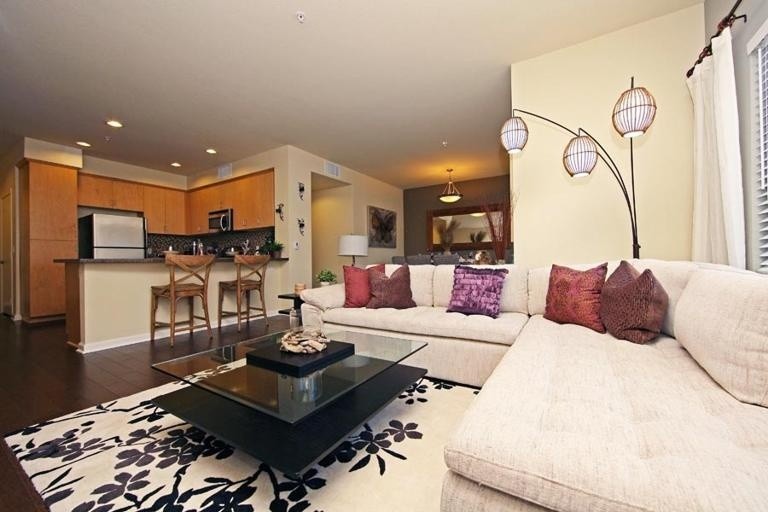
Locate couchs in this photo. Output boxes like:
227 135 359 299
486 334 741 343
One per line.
300 258 768 512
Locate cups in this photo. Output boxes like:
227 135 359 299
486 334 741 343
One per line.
289 309 322 340
290 372 324 403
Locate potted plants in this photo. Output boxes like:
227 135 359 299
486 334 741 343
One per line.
314 266 338 287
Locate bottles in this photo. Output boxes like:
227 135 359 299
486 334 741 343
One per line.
148 239 152 256
192 239 205 256
255 242 260 256
169 245 172 251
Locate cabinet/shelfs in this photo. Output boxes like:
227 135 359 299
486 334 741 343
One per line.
143 183 185 237
77 172 143 211
21 241 80 320
206 181 231 212
185 184 211 237
18 164 78 241
232 169 274 229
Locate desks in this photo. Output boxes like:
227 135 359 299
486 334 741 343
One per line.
278 286 321 320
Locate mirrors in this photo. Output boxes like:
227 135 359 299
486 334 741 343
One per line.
425 203 511 251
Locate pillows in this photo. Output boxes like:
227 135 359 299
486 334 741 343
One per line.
365 263 418 308
600 259 670 344
342 264 386 307
542 261 610 335
445 264 509 318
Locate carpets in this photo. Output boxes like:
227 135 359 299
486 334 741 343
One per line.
4 350 482 512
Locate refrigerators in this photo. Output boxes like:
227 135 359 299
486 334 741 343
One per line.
79 213 147 258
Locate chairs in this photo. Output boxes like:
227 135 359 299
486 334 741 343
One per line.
219 254 270 331
391 247 514 265
149 252 217 348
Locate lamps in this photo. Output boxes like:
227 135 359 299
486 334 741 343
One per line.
437 168 463 203
499 77 657 259
338 234 369 266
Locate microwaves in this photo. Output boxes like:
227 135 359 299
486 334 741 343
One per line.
208 208 231 234
